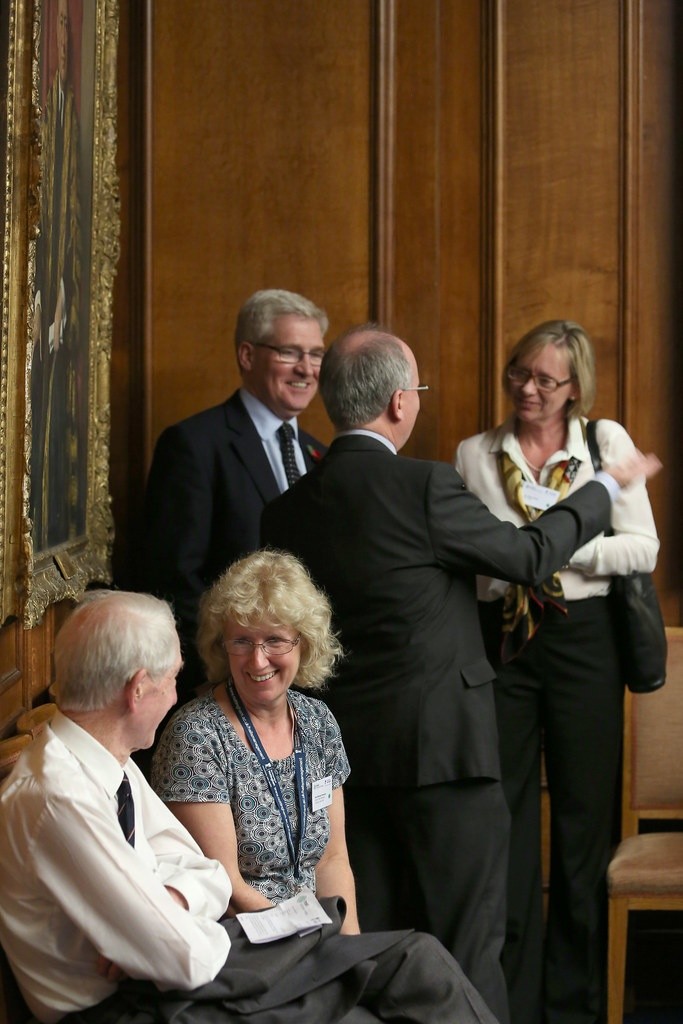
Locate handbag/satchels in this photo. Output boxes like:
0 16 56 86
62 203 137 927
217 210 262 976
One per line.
608 573 668 693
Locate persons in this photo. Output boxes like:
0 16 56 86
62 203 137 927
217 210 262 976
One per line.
134 288 331 686
0 591 500 1023
259 321 663 1024
149 545 361 936
454 322 660 1023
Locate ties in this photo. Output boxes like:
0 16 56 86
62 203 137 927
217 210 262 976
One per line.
59 92 62 128
116 770 135 848
275 422 301 488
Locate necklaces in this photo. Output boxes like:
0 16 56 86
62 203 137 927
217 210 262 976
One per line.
524 456 544 473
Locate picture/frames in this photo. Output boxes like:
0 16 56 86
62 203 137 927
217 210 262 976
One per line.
0 0 123 624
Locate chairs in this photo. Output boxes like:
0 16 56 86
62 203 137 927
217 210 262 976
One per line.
603 627 683 1024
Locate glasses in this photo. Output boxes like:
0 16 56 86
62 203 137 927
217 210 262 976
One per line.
251 341 325 366
59 15 70 31
222 632 302 657
389 384 428 404
507 366 575 393
